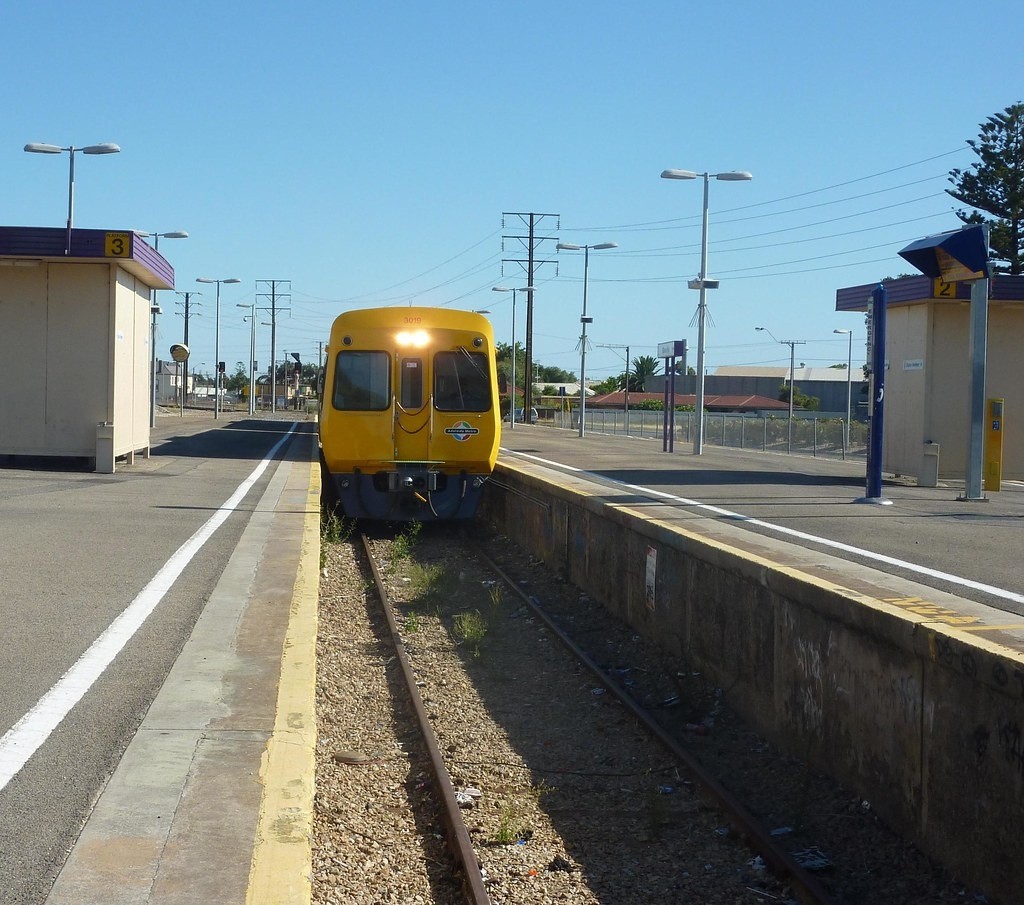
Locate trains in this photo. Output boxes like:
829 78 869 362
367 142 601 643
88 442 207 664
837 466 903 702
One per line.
316 306 501 536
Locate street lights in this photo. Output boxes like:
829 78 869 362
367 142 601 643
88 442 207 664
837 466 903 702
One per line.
24 143 121 229
834 329 852 451
196 278 241 419
492 287 538 428
662 169 753 455
556 243 618 437
129 229 189 427
236 303 255 416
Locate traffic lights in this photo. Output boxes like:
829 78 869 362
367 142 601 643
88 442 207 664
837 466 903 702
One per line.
219 362 225 372
295 362 301 374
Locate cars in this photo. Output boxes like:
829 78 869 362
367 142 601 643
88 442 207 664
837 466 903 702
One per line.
503 407 538 425
